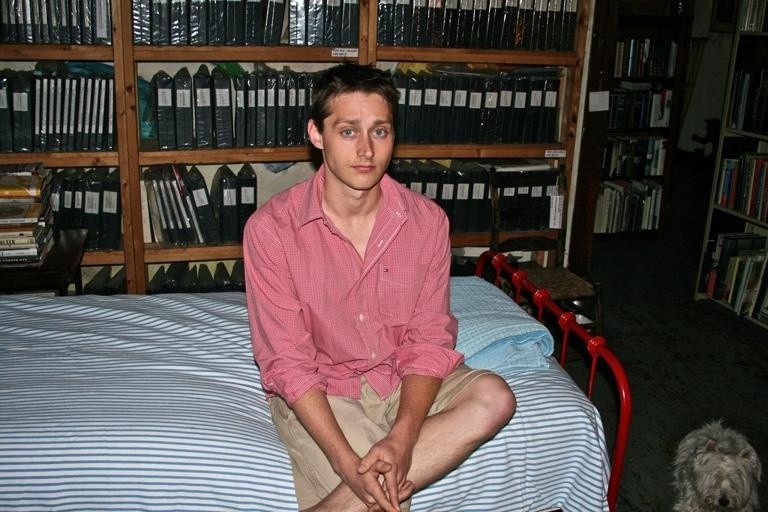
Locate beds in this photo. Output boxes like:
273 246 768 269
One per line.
1 251 632 511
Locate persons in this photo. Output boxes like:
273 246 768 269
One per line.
243 64 515 512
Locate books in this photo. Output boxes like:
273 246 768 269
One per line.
0 163 56 269
594 36 678 235
699 0 768 322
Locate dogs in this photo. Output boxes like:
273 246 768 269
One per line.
668 417 763 512
